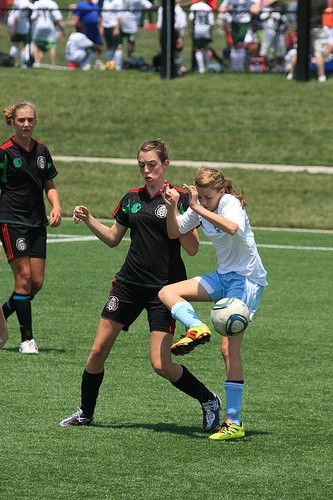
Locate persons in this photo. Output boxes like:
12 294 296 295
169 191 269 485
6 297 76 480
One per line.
0 101 62 355
0 0 333 84
58 138 223 432
157 167 269 440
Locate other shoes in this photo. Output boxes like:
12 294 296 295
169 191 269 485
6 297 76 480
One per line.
231 64 244 73
32 62 40 68
95 60 106 71
274 63 284 72
82 63 91 72
115 64 122 71
249 64 270 72
21 62 28 70
287 72 293 80
199 66 206 74
318 74 327 83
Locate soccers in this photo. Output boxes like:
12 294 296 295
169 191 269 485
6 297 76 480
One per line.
210 297 250 337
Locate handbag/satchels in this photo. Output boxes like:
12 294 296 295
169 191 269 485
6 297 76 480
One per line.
0 52 14 67
122 54 184 74
223 50 287 68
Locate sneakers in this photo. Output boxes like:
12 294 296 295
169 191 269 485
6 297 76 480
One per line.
60 407 95 427
19 338 38 354
170 323 212 356
201 392 222 432
208 418 245 440
